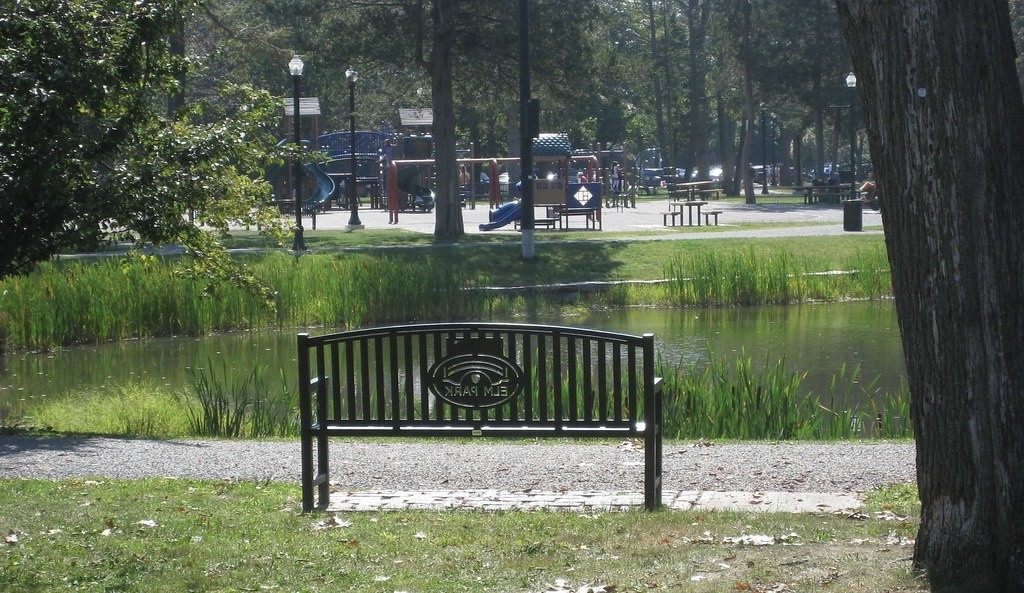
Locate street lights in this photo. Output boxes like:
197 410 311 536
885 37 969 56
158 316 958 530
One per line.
288 55 309 250
759 103 769 194
842 71 865 232
770 113 777 186
345 71 365 229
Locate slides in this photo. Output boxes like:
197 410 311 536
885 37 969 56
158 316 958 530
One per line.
479 203 522 231
398 163 435 210
299 159 335 213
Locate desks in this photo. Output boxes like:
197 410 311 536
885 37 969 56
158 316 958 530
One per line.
669 202 708 226
676 181 713 200
802 186 842 202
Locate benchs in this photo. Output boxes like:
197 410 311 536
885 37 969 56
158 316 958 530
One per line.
693 189 725 199
660 211 683 226
700 211 722 225
804 193 840 203
297 322 663 517
669 188 700 201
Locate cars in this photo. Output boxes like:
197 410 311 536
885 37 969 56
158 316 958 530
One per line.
643 168 686 186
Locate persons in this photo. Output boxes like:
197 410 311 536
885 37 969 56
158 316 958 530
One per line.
578 172 587 182
460 165 470 203
767 164 780 185
339 184 345 203
611 160 624 191
377 138 393 168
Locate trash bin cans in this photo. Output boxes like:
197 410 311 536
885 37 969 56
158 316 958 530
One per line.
840 198 864 231
